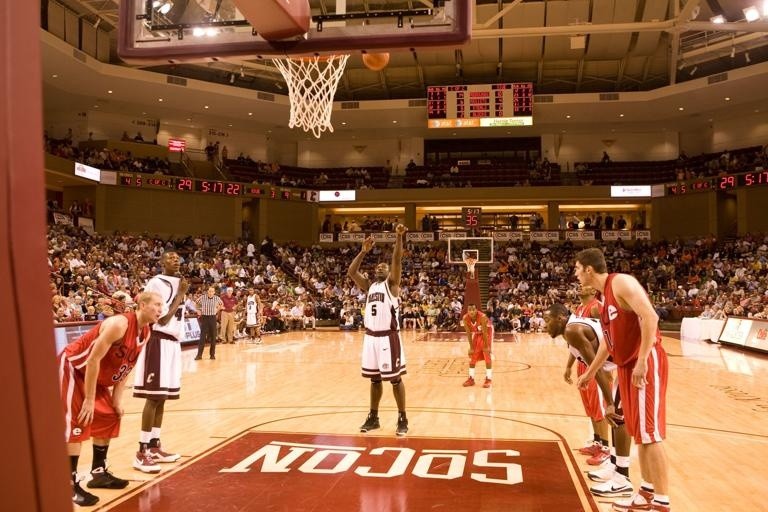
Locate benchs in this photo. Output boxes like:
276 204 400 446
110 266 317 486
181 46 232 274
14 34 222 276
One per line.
576 146 764 185
404 158 561 188
233 160 387 190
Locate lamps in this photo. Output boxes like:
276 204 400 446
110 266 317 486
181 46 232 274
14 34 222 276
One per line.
158 1 174 14
743 5 761 23
710 14 725 24
192 16 219 36
571 36 586 49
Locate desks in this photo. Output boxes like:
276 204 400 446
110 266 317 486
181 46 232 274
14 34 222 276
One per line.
680 318 726 343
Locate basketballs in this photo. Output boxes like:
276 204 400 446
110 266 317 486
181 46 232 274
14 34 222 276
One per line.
363 52 390 71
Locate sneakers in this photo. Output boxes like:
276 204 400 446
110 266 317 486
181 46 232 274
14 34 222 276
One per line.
148 443 180 463
87 462 128 489
483 376 492 388
579 440 671 512
360 413 380 432
395 418 408 436
70 476 100 507
463 376 475 387
133 449 161 473
246 337 262 344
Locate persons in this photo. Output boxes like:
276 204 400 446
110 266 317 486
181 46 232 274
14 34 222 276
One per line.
348 223 409 436
462 304 494 389
194 286 224 360
43 133 768 345
544 279 635 498
574 247 670 512
133 251 189 474
59 291 163 507
